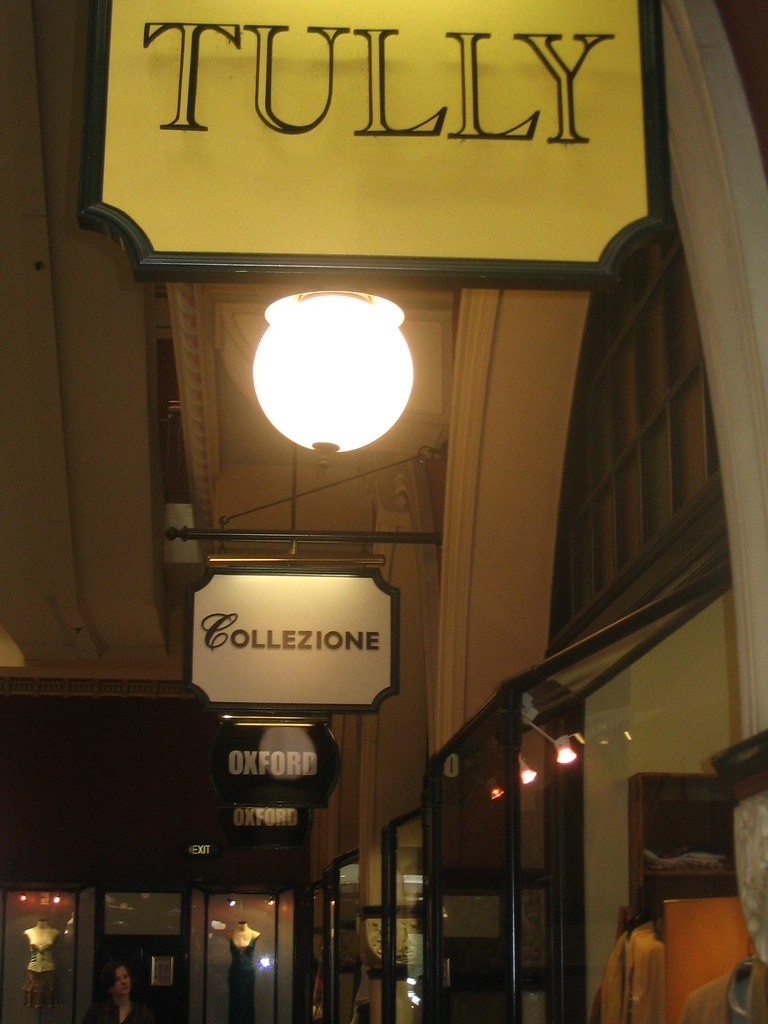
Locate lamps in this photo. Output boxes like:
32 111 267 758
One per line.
53 893 62 904
266 895 276 906
251 294 415 471
519 751 538 785
522 693 577 764
226 895 237 907
484 778 504 800
19 892 28 902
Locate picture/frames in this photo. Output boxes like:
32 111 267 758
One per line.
151 956 174 986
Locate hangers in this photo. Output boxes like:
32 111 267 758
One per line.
623 886 648 939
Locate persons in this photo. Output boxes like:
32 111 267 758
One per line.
23 919 64 1007
81 960 155 1024
224 921 260 1024
